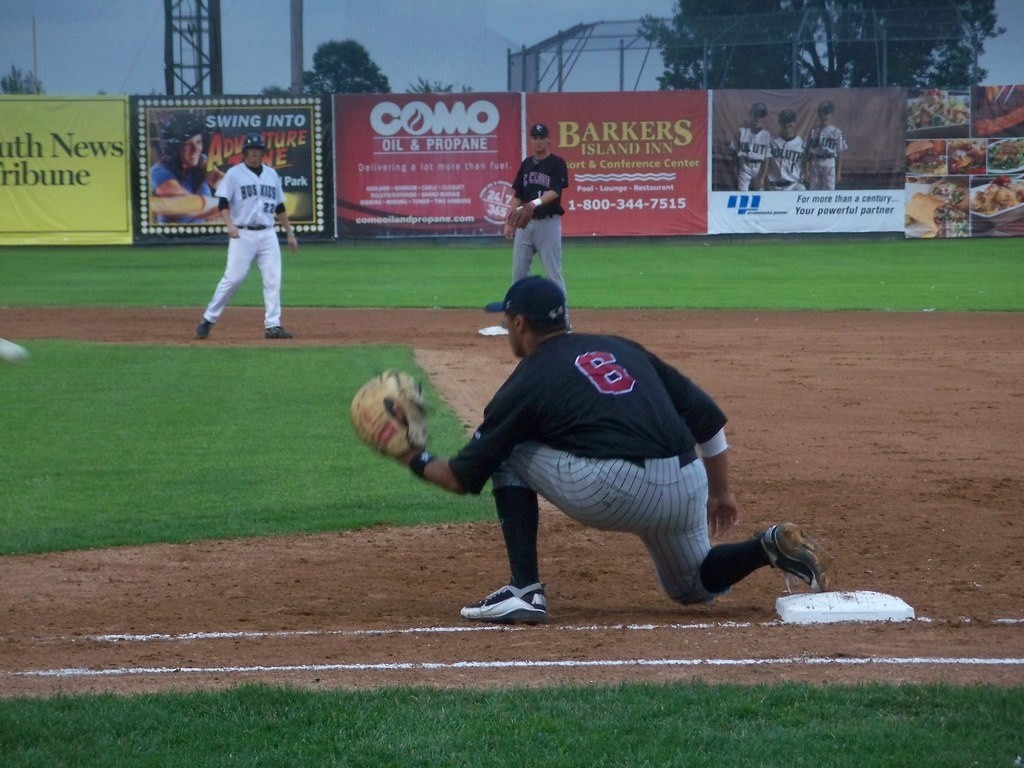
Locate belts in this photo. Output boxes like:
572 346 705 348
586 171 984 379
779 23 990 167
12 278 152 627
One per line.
817 155 830 159
771 180 791 186
237 225 265 231
746 159 760 163
532 214 553 220
625 447 698 468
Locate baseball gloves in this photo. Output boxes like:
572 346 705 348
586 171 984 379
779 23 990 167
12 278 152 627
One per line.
506 203 535 229
347 369 427 464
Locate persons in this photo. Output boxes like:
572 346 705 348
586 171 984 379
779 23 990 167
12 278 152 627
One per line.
766 108 808 191
729 103 771 192
501 124 571 335
150 112 225 224
802 100 848 190
350 275 824 622
196 133 299 339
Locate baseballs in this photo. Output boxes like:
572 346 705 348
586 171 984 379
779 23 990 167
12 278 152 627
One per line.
0 338 26 364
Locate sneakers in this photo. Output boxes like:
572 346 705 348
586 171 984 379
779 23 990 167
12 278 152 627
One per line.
753 523 833 593
197 318 213 339
265 326 293 339
460 580 546 623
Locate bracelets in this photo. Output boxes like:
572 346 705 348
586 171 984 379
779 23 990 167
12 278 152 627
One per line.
287 233 294 237
409 451 434 477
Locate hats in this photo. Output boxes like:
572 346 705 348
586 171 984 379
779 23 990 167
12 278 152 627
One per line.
777 109 797 124
530 123 549 136
484 275 568 323
749 103 767 118
816 100 836 113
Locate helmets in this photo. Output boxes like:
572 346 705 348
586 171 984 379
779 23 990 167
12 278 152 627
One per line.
159 112 208 163
242 132 264 148
